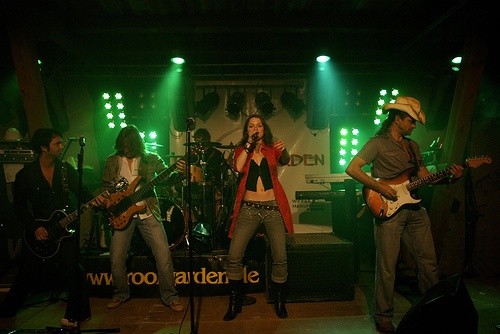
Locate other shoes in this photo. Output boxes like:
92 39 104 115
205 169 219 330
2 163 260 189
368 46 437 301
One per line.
376 322 394 334
105 296 130 310
169 299 184 312
60 318 80 329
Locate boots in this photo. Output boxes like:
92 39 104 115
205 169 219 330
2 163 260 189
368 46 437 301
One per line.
223 278 245 321
271 279 289 319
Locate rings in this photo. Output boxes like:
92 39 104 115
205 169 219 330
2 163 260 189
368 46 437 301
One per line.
181 167 183 171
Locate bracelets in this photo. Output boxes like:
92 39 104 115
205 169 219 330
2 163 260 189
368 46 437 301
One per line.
247 141 252 145
448 175 456 184
244 146 253 153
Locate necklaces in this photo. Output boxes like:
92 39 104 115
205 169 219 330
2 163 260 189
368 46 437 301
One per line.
391 135 412 161
255 142 262 145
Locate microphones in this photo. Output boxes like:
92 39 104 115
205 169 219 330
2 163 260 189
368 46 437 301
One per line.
79 135 87 147
253 132 258 139
186 117 195 123
68 136 80 142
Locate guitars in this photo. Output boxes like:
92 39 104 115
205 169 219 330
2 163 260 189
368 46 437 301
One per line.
363 154 492 222
104 143 205 231
25 175 130 260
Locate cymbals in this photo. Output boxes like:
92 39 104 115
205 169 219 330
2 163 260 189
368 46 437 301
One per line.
163 154 185 158
182 140 222 149
217 144 237 150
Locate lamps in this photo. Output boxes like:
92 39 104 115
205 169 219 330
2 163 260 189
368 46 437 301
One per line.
194 74 306 117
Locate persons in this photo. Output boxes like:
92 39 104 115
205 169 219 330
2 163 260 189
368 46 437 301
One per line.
223 115 294 321
345 97 465 334
192 128 227 185
103 124 188 312
0 128 107 326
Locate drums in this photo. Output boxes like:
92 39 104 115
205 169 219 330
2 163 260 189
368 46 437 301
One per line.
174 163 207 194
133 196 195 250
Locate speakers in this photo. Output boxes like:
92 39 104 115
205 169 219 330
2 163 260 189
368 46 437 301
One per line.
79 202 107 250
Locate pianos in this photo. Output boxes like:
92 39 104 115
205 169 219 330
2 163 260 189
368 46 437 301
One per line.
294 173 372 233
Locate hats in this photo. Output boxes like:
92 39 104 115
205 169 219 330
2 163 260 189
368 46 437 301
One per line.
382 96 426 125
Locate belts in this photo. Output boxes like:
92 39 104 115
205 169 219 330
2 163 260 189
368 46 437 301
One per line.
242 200 279 211
